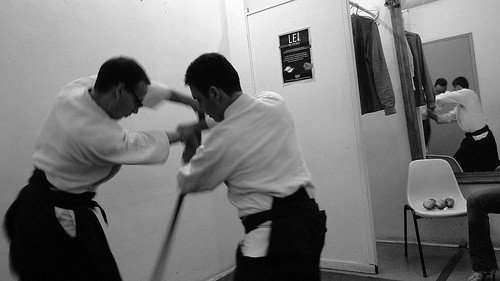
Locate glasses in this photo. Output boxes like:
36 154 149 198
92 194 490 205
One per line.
131 90 143 107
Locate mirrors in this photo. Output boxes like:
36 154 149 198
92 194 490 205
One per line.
398 1 500 184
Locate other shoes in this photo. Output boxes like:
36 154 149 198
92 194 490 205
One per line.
466 266 500 281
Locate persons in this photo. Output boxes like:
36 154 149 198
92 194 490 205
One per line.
467 186 500 281
177 52 328 281
430 76 500 171
3 56 203 281
419 76 448 146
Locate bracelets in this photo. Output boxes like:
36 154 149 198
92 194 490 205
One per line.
177 127 186 141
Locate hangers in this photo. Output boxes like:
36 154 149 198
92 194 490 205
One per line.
350 3 380 27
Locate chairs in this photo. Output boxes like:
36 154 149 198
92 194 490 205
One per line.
404 159 490 277
